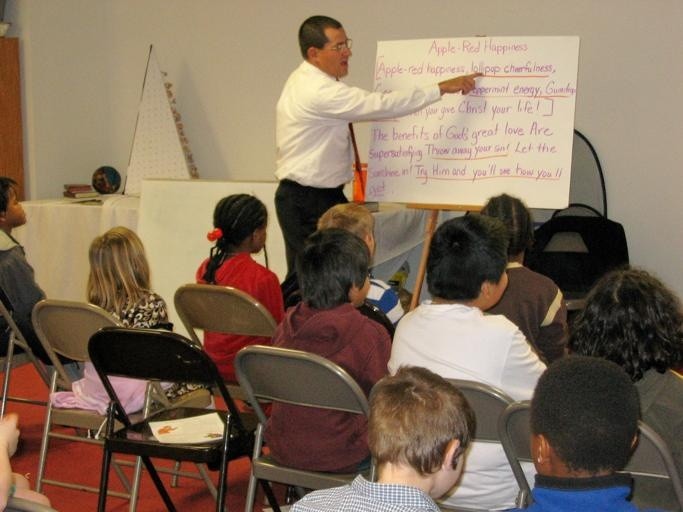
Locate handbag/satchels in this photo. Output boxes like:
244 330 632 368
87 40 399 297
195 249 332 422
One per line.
522 204 629 296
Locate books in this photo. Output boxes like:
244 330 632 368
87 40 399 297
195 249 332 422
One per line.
63 183 99 200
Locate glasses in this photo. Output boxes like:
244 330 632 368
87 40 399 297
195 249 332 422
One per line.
320 39 352 50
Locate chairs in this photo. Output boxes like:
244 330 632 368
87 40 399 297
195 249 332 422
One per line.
234 345 378 511
498 401 683 511
433 378 516 512
33 301 228 511
171 284 279 488
88 326 292 512
1 301 85 437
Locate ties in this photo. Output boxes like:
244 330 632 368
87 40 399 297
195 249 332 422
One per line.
336 77 366 195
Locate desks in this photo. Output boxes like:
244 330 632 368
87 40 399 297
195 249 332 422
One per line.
11 193 140 303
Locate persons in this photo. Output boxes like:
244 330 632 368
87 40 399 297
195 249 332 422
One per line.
48 225 215 419
261 195 682 511
266 15 482 309
0 176 84 390
0 412 57 512
194 193 287 412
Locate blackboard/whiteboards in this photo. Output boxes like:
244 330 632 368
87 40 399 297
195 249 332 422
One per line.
139 178 288 347
365 36 581 210
